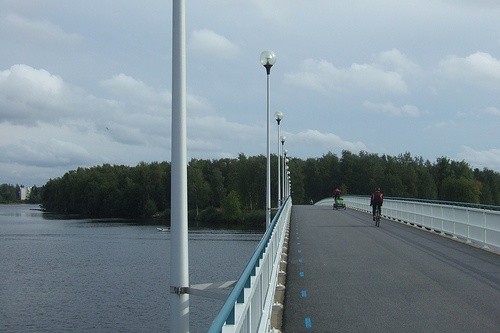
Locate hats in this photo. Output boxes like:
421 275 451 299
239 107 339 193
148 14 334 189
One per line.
376 187 381 191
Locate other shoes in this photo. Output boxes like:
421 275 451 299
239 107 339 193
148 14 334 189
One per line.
373 217 375 221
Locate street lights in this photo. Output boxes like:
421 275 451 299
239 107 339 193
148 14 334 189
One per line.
260 49 276 231
280 135 287 202
283 148 291 200
274 111 283 207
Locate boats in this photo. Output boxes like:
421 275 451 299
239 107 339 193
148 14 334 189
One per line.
156 227 171 232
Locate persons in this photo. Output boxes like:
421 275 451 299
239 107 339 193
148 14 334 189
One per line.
333 189 343 200
370 188 383 221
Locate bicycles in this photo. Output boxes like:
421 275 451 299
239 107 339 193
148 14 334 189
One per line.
371 201 383 227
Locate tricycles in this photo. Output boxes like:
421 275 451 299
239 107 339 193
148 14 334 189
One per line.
332 194 346 211
